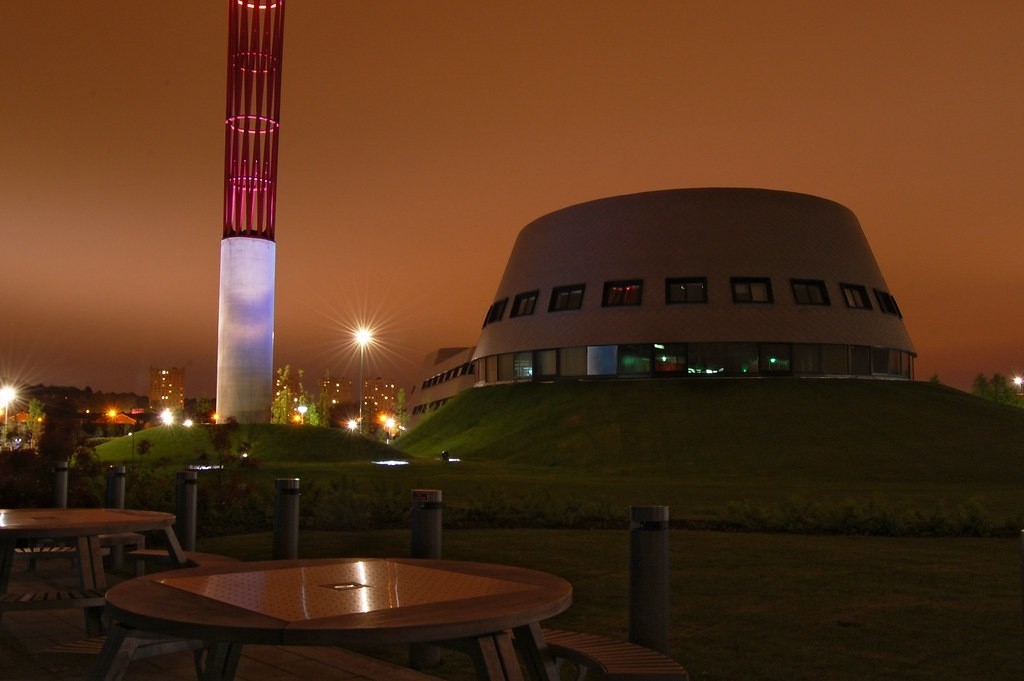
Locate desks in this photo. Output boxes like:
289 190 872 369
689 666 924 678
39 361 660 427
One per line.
84 557 574 681
0 507 187 641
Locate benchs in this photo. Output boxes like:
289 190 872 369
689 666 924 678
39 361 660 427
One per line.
0 588 106 613
13 545 244 566
505 629 688 681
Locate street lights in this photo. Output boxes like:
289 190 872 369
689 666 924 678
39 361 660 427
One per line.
2 384 13 446
352 324 372 434
